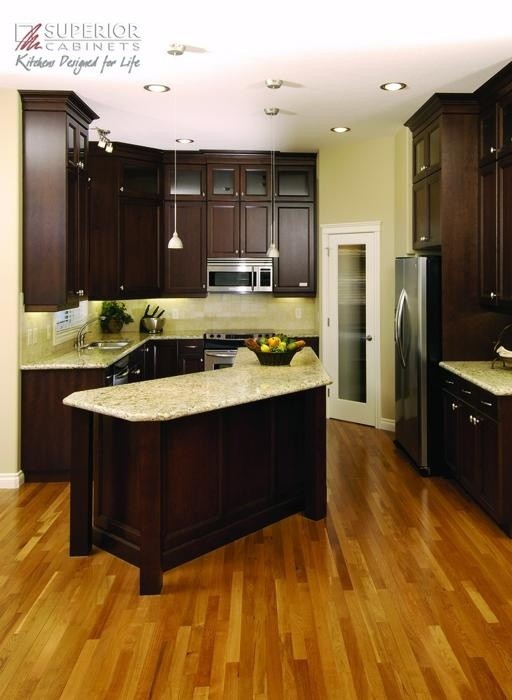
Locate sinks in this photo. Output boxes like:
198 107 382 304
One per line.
80 340 131 350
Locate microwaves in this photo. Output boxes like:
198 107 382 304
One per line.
206 257 274 295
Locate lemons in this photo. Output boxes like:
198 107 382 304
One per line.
257 337 305 352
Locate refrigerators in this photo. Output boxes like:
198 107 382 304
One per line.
392 255 444 476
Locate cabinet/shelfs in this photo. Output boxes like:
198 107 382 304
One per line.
161 150 206 299
207 152 274 258
149 340 176 379
89 140 160 302
439 367 512 541
403 92 471 252
19 89 99 312
129 345 145 383
273 152 318 296
473 62 511 311
178 341 203 374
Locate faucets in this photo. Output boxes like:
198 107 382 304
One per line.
77 316 106 347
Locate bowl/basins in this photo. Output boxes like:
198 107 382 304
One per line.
142 317 167 334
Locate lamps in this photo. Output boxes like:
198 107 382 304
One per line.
168 149 184 250
96 127 113 154
265 152 280 258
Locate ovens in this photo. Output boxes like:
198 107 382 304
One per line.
204 352 239 371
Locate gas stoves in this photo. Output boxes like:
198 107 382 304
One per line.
204 328 276 350
